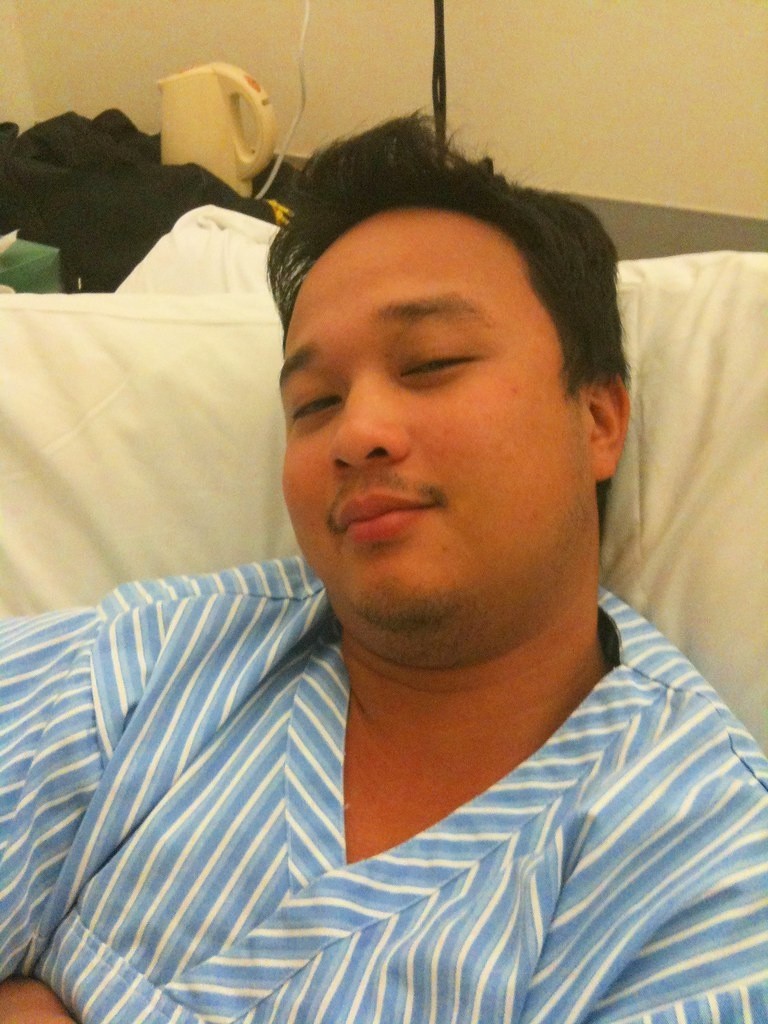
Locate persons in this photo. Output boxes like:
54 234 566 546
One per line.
0 114 768 1024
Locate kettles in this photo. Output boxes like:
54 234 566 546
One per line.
155 62 277 199
